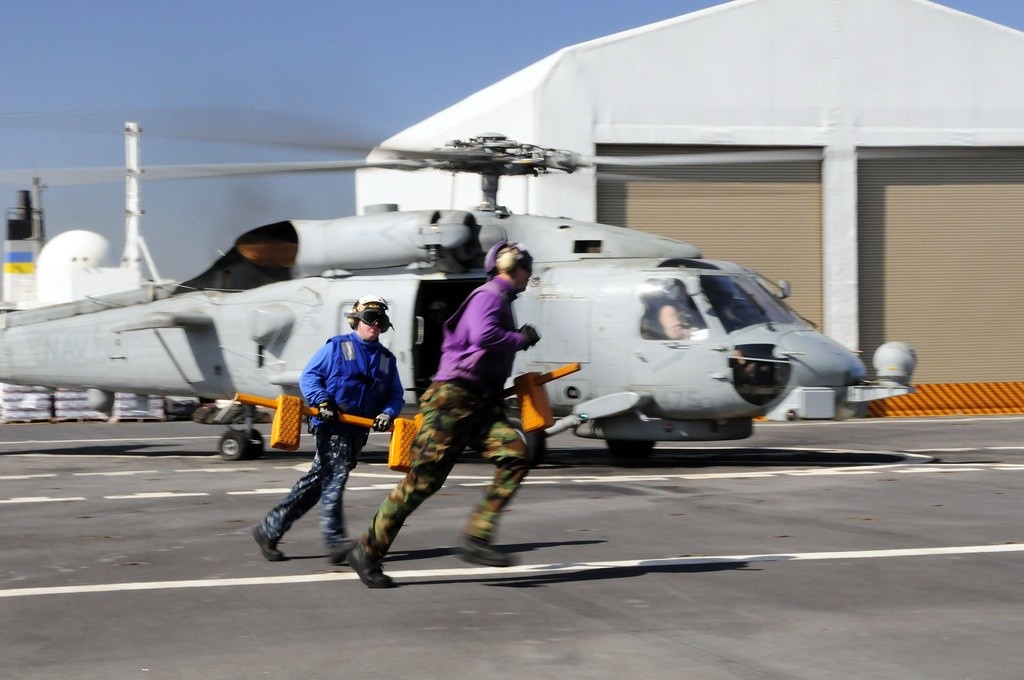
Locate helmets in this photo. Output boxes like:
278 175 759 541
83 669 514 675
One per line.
484 240 526 277
345 294 388 328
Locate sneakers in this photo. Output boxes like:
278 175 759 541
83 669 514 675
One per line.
461 537 509 566
253 525 287 561
329 538 362 563
346 547 390 589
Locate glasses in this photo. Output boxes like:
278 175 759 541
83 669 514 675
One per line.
360 310 390 330
517 252 533 272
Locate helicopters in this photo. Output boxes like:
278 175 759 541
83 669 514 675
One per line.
1 115 921 469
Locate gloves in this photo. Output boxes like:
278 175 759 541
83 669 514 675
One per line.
373 413 392 433
318 402 340 423
519 324 539 351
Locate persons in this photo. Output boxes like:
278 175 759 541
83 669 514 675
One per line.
254 294 405 567
653 282 706 340
348 239 534 584
